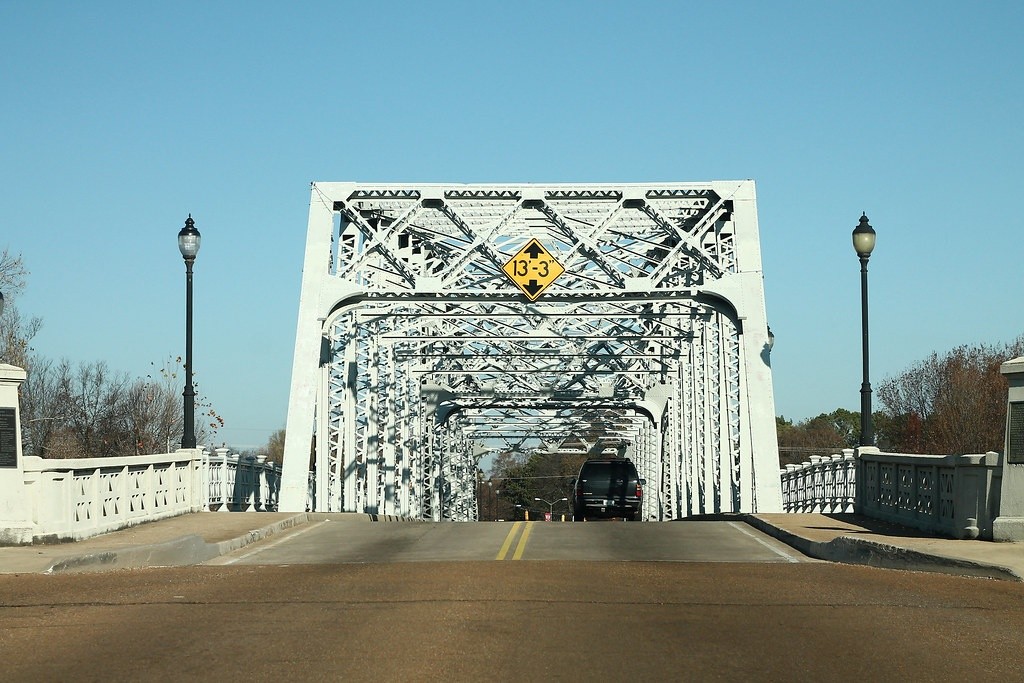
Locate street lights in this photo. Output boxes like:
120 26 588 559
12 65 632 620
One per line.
852 210 878 447
176 213 203 448
534 496 569 522
495 489 500 521
478 467 485 521
487 478 493 521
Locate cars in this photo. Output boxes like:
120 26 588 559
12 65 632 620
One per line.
570 457 646 521
